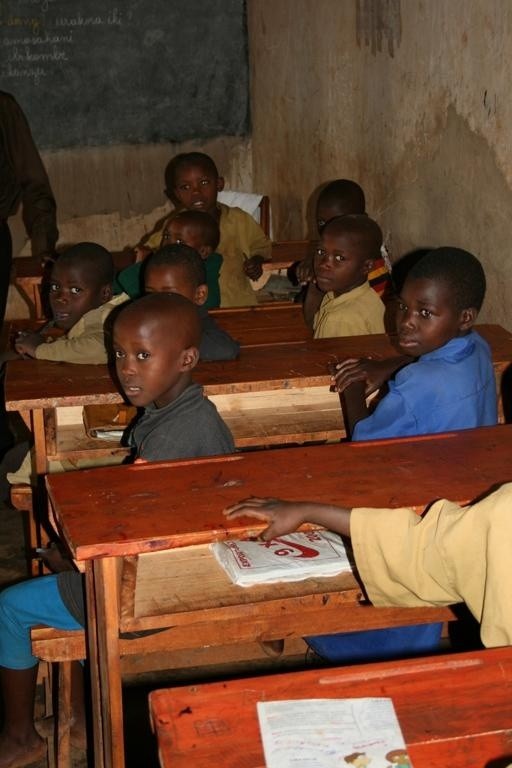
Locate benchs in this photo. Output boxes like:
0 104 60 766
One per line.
30 599 457 762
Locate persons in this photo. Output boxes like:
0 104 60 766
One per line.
302 213 384 341
0 291 235 767
255 246 496 669
223 361 512 649
0 88 59 327
139 150 275 307
1 241 129 484
289 178 389 307
138 244 239 362
111 210 222 311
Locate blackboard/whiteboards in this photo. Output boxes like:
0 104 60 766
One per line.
0 0 250 150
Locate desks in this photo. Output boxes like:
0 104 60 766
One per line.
4 324 512 576
40 296 319 348
44 423 512 768
148 644 512 768
12 238 321 287
